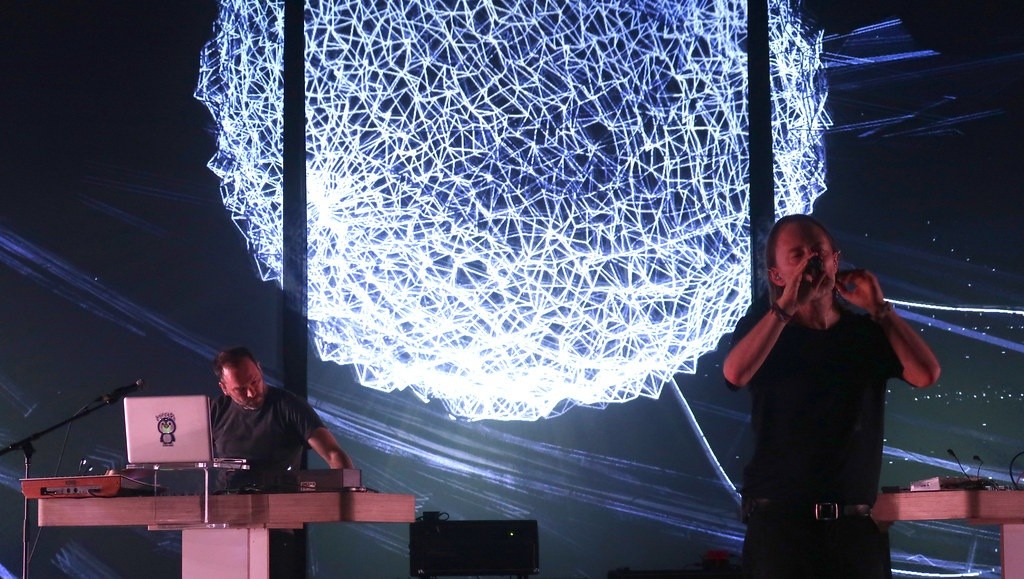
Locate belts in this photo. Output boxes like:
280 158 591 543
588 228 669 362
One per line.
755 497 871 522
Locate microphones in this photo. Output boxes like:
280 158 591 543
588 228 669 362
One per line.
97 379 144 403
808 256 824 277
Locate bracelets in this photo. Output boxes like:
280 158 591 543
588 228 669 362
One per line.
872 301 893 322
771 302 792 321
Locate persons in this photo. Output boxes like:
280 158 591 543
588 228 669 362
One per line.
106 346 378 579
718 214 941 579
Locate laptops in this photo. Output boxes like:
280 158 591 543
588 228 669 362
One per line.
123 394 246 464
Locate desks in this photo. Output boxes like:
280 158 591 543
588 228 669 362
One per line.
873 490 1024 579
126 461 249 529
38 493 414 579
